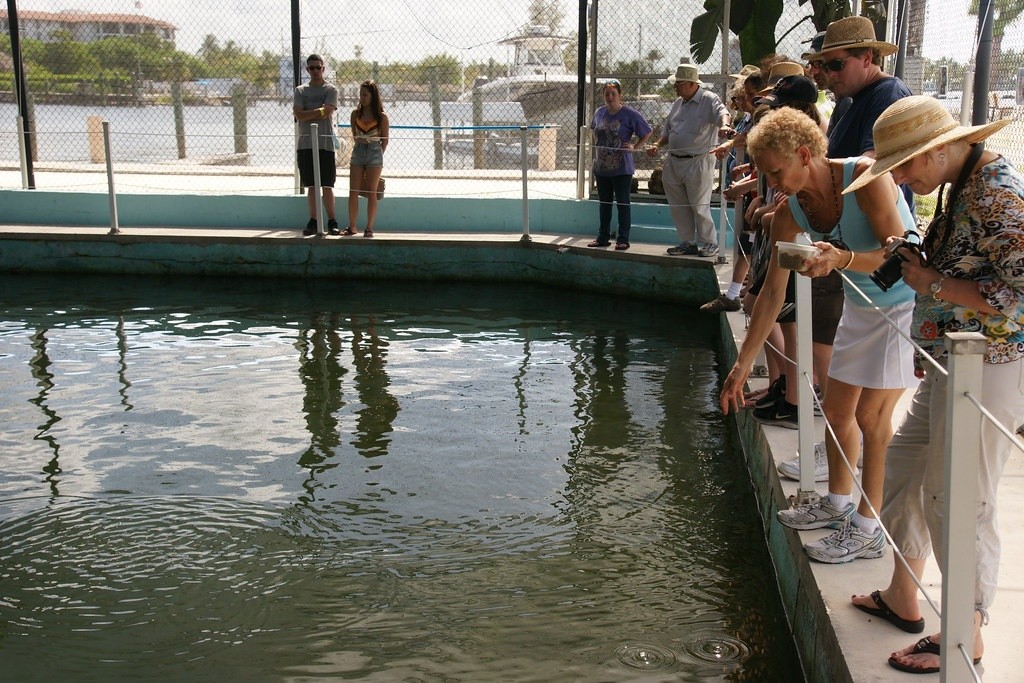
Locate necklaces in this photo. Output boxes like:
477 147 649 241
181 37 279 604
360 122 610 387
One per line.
803 158 845 250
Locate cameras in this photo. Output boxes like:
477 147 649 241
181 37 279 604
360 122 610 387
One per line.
869 238 922 292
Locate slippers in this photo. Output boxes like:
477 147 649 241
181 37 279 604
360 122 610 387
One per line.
743 388 770 408
888 635 982 675
339 227 373 237
587 241 630 250
852 590 925 633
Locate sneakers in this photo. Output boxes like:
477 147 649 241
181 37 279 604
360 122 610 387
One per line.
698 242 720 257
778 438 863 485
749 372 824 430
801 515 888 564
327 219 340 235
303 217 317 235
666 241 698 256
699 293 742 315
777 496 857 530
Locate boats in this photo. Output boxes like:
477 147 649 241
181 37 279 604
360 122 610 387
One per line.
441 132 546 163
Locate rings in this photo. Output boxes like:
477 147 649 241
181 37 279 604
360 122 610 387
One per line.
816 274 819 277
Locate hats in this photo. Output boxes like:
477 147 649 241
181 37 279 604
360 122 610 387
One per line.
729 62 818 107
801 16 898 63
667 64 704 85
840 95 1011 197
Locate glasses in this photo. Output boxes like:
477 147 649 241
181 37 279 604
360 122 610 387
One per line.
805 54 857 72
308 65 322 71
605 80 618 84
675 81 687 85
731 97 739 102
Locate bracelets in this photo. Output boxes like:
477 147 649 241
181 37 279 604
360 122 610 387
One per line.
654 143 660 147
319 107 325 116
841 250 854 270
727 140 734 148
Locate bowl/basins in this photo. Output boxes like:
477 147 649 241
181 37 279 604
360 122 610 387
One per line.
775 241 823 272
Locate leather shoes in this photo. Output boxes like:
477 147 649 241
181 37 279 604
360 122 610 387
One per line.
670 153 700 159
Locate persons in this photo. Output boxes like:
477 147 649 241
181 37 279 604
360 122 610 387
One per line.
655 16 1024 672
293 54 340 236
340 79 389 237
587 80 653 250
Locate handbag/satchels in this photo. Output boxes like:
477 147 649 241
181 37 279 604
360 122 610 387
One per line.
357 178 385 201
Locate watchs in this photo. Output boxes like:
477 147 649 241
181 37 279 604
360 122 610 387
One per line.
929 273 949 302
